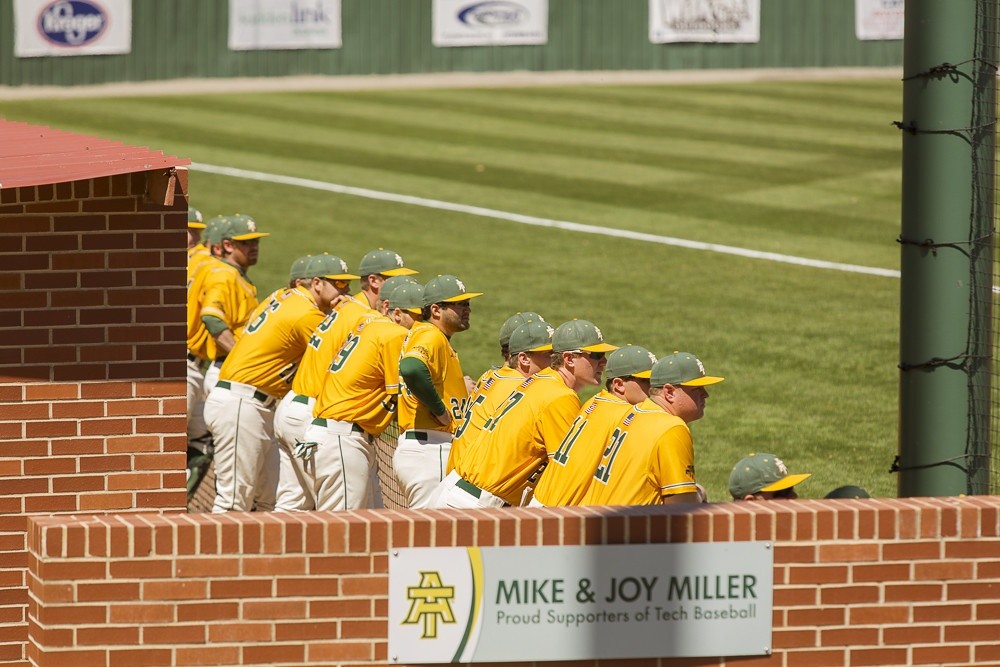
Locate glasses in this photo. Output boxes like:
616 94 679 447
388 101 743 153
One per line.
319 277 351 288
563 350 605 361
767 485 794 500
437 300 470 309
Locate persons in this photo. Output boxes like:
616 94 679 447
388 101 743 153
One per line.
185 207 724 526
728 452 810 505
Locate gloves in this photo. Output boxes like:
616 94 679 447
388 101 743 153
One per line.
291 438 318 461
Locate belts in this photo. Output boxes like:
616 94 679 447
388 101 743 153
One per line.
214 360 224 368
215 381 276 407
292 395 308 405
455 479 512 508
406 432 427 440
311 418 374 443
187 353 205 368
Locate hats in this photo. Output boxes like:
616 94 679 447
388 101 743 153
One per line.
509 320 555 355
188 207 207 229
498 312 545 346
379 276 419 301
202 214 226 238
551 318 620 352
388 282 424 314
221 213 270 240
605 343 658 379
650 351 724 387
209 219 228 244
305 252 361 280
359 248 420 276
290 255 316 279
728 453 812 499
422 275 483 308
